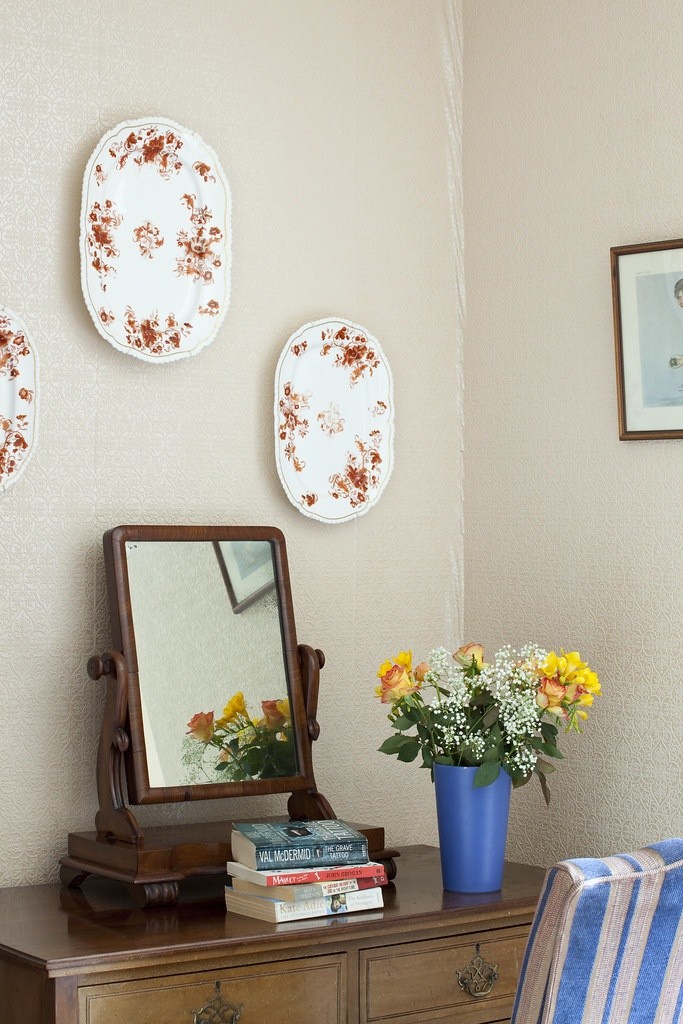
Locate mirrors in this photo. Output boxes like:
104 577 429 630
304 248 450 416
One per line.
58 526 401 914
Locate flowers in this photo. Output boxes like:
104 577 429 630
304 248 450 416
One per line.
374 640 603 808
178 691 300 787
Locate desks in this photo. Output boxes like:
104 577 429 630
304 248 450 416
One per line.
0 843 548 1024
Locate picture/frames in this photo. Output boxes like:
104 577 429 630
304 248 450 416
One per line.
609 237 683 442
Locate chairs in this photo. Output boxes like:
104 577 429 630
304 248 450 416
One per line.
512 838 683 1024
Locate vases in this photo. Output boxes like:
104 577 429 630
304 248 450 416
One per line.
433 762 512 894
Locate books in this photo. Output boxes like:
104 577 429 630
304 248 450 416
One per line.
231 874 388 903
230 818 370 871
224 886 384 925
226 860 385 887
